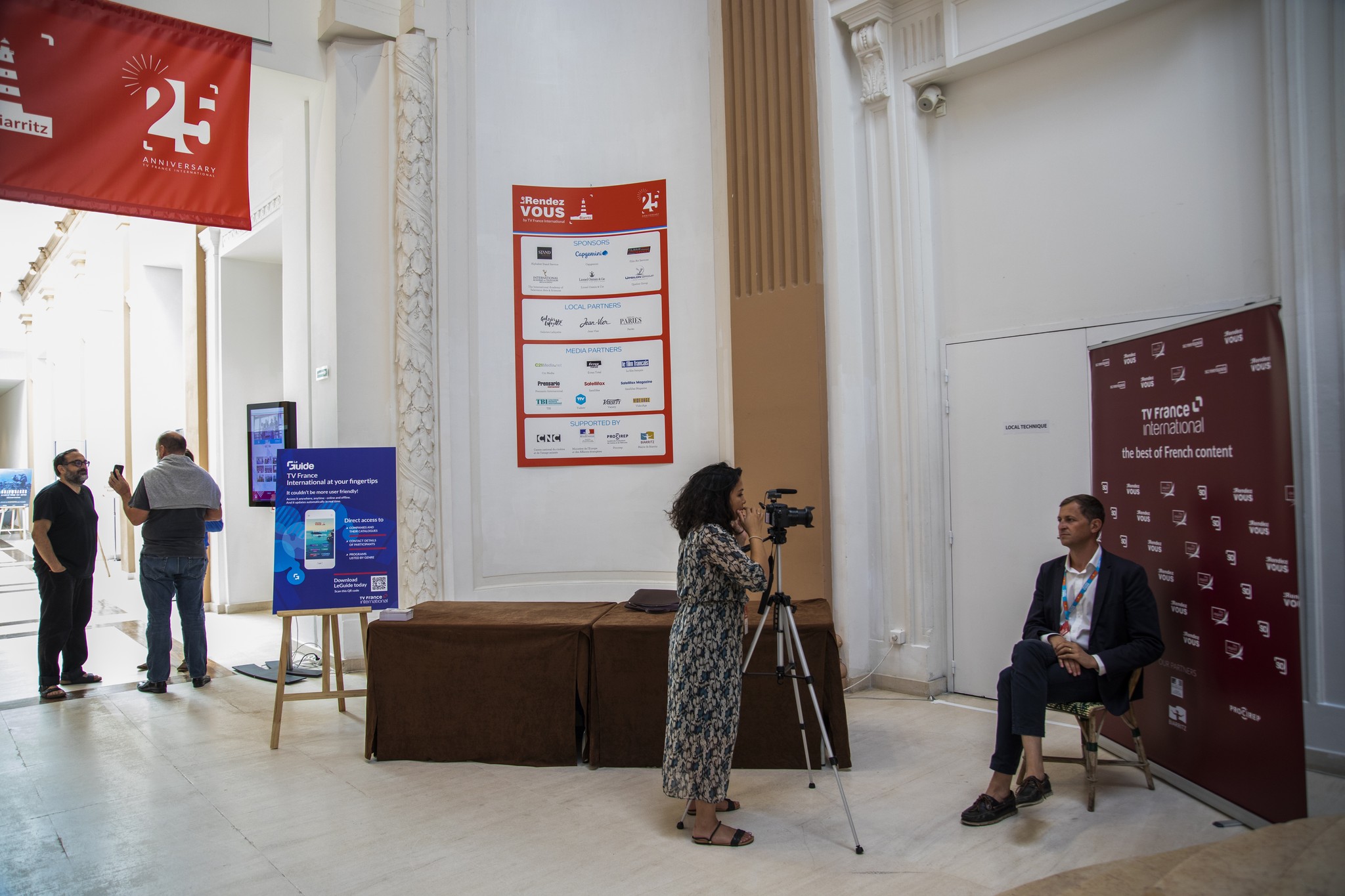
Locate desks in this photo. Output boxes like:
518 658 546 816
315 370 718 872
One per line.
363 600 618 769
589 597 853 771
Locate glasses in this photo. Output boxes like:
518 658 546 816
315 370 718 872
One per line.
61 460 90 468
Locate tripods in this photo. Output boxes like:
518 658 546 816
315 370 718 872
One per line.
676 531 866 853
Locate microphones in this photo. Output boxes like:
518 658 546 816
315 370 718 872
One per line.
767 489 798 494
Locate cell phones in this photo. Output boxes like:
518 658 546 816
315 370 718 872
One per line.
113 465 124 480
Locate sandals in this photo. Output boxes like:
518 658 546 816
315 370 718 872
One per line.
692 821 754 846
38 686 67 699
60 673 102 685
686 799 740 815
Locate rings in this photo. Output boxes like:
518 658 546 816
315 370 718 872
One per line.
757 512 761 515
1068 648 1072 653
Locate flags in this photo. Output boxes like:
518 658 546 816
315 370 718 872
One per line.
1 0 255 236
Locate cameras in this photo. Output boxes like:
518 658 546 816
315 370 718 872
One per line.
758 502 816 529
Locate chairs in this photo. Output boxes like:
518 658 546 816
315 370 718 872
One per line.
1014 666 1156 812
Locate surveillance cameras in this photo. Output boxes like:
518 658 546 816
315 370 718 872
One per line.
917 85 946 113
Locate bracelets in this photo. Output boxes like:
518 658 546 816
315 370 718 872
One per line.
749 536 763 542
733 529 744 534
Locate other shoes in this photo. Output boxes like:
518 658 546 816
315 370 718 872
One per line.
177 659 189 670
136 663 148 670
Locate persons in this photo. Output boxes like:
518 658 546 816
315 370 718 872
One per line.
960 493 1165 827
663 461 770 848
30 449 104 698
108 429 222 694
135 448 224 672
254 414 280 482
17 473 27 489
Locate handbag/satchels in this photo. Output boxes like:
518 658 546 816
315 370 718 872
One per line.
624 589 680 614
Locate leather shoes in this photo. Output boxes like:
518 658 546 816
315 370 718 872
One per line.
961 790 1018 826
1015 774 1052 808
192 674 211 687
137 679 167 694
